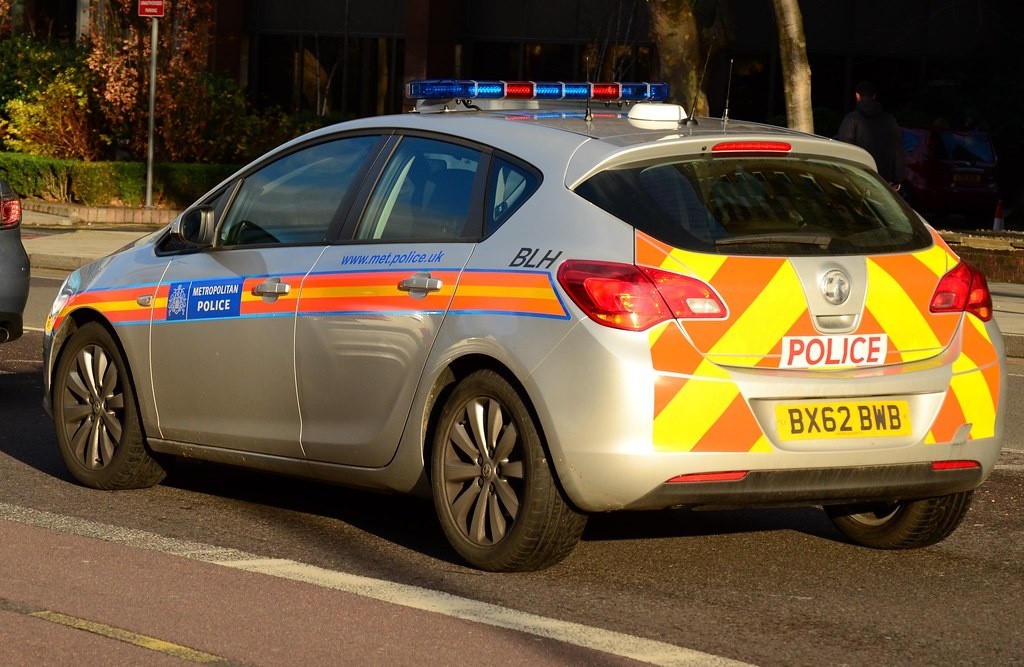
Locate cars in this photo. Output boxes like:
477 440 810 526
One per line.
0 176 31 344
41 76 1008 571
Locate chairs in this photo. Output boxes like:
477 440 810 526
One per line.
411 170 475 237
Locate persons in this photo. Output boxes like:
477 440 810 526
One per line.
931 79 1004 214
839 81 906 193
